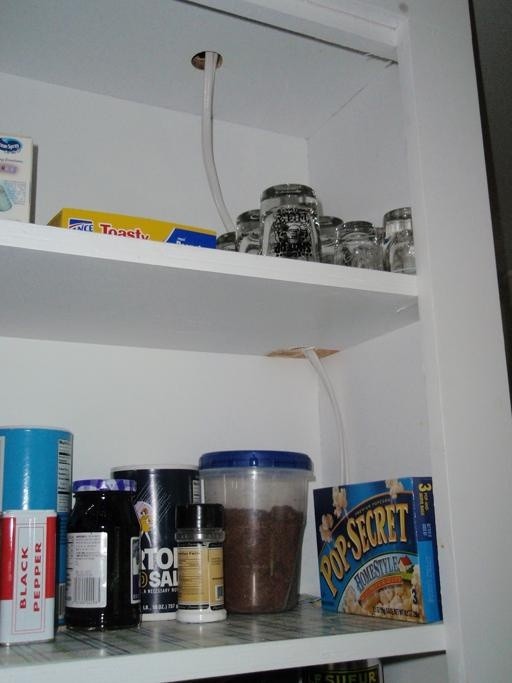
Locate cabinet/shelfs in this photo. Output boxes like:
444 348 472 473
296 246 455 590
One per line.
0 0 512 683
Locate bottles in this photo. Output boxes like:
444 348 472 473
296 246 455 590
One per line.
173 503 227 624
112 464 201 621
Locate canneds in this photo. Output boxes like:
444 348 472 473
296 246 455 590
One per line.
216 184 417 276
68 478 140 630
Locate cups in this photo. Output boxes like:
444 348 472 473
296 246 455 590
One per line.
200 449 314 617
215 184 414 275
